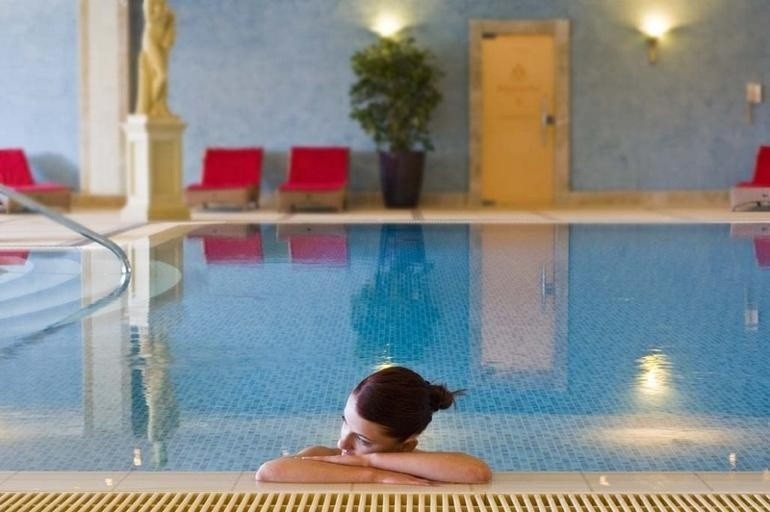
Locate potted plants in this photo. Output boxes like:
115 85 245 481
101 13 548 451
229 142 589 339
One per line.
348 37 446 208
350 224 442 367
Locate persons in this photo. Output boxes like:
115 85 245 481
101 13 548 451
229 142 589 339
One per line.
252 365 492 488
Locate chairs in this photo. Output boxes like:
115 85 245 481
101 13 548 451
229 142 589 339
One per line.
186 224 264 264
731 225 770 269
274 145 351 214
730 146 770 214
1 250 29 266
278 225 349 268
181 146 262 213
0 148 74 215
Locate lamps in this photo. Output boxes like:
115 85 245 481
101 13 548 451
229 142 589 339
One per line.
642 19 669 63
